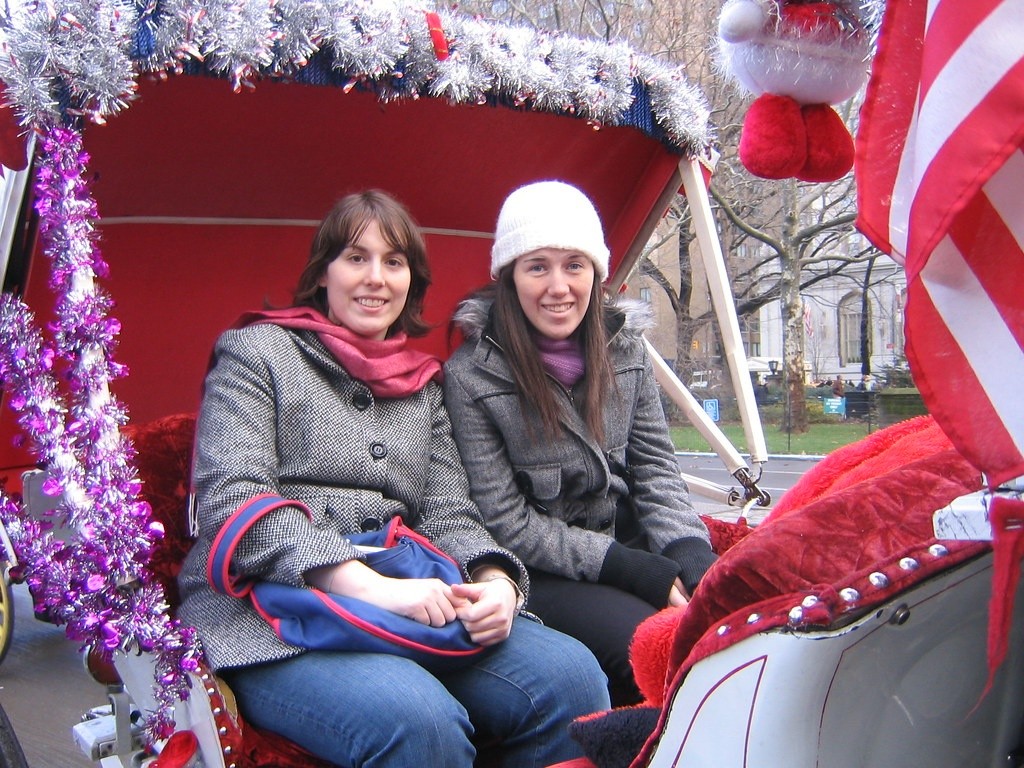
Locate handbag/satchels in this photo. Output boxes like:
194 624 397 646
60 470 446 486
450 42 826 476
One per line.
206 496 489 671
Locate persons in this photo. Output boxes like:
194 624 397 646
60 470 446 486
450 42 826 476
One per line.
816 374 886 399
445 181 721 707
175 189 612 768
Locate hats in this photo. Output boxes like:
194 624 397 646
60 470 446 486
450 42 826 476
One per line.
489 182 610 284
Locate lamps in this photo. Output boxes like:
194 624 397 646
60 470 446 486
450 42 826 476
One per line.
768 359 778 374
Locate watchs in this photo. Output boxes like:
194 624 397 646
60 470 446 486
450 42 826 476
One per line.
487 574 525 617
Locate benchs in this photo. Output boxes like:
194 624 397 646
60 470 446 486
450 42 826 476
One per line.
79 412 754 768
